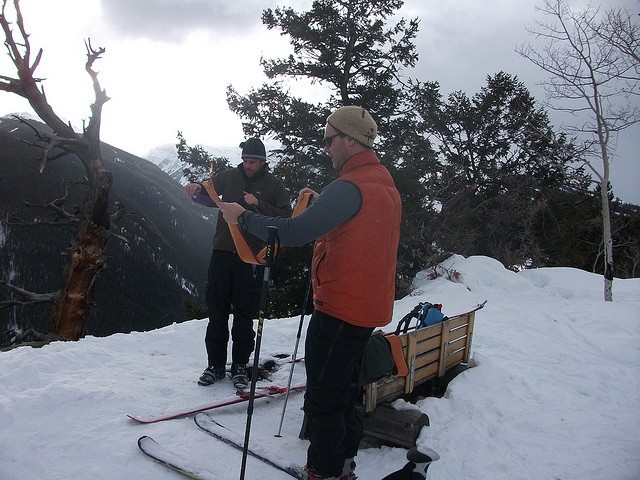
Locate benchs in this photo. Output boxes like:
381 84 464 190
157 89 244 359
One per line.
329 308 476 448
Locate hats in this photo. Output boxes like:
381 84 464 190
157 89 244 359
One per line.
326 105 377 149
240 137 266 160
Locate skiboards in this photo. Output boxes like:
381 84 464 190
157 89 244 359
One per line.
138 412 293 480
127 299 488 424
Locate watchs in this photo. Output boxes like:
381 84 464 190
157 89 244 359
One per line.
237 210 248 230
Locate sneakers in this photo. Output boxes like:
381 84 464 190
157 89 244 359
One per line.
339 471 358 480
304 463 333 480
197 364 226 386
230 361 249 390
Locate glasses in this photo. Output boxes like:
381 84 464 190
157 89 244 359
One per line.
322 133 342 147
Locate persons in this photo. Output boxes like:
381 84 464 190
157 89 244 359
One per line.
214 103 403 480
185 137 292 389
